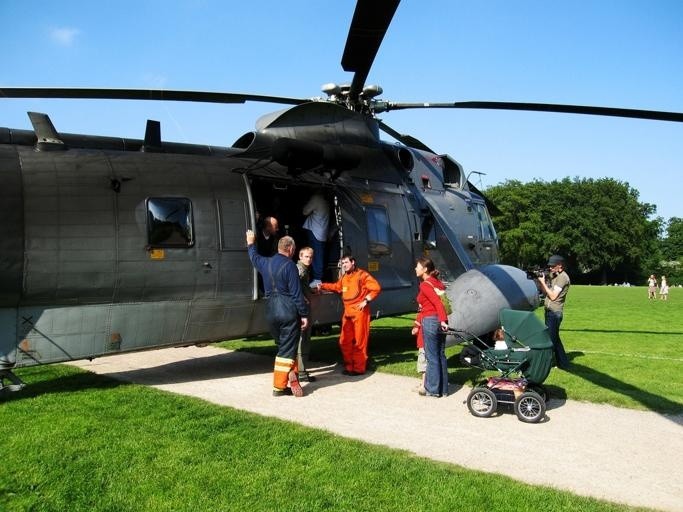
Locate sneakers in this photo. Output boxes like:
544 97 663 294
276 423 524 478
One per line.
308 279 322 288
342 370 360 376
271 369 315 397
419 388 448 398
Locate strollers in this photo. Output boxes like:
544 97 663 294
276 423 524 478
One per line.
438 308 555 423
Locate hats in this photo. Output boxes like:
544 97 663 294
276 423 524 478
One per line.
547 255 565 265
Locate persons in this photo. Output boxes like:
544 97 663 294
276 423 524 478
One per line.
300 188 332 289
307 252 382 379
410 303 431 396
292 246 317 383
253 210 281 258
647 274 658 299
532 254 571 370
244 228 310 397
413 256 451 398
659 275 670 300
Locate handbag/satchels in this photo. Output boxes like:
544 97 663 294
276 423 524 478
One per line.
424 280 452 315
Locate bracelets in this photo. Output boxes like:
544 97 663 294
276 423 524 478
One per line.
363 297 369 304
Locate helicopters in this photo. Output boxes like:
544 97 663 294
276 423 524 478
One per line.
1 0 683 414
